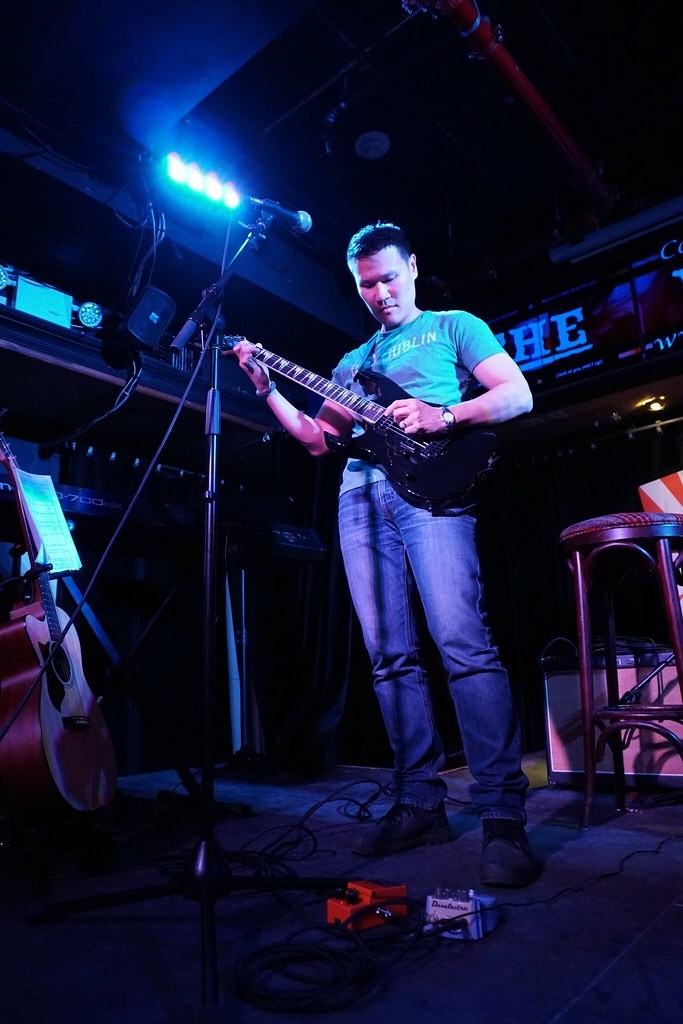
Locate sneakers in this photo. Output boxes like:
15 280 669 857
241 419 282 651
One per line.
353 800 451 857
478 818 536 885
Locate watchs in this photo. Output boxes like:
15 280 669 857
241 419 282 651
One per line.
439 406 456 434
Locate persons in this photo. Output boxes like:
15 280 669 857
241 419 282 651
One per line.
231 220 541 890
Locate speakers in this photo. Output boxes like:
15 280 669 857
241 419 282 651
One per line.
539 649 683 789
118 286 177 350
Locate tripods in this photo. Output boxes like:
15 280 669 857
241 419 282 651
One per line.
50 211 364 1024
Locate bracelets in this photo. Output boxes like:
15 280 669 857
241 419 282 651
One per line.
255 382 276 397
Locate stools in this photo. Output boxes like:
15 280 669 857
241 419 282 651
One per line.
559 510 683 830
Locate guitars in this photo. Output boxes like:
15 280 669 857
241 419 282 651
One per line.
219 334 505 510
0 429 118 813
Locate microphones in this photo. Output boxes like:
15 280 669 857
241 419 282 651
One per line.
618 687 641 705
243 197 313 234
